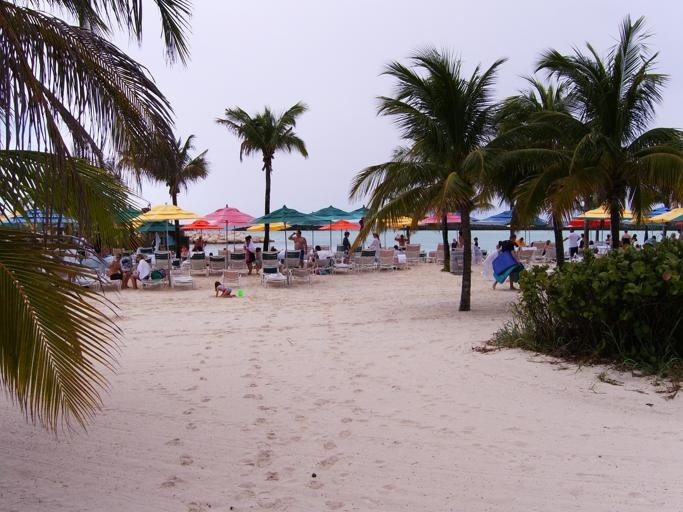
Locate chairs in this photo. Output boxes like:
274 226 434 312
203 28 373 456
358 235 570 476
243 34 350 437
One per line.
336 239 610 277
53 238 335 287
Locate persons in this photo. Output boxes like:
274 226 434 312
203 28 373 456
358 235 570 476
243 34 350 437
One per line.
213 281 235 299
